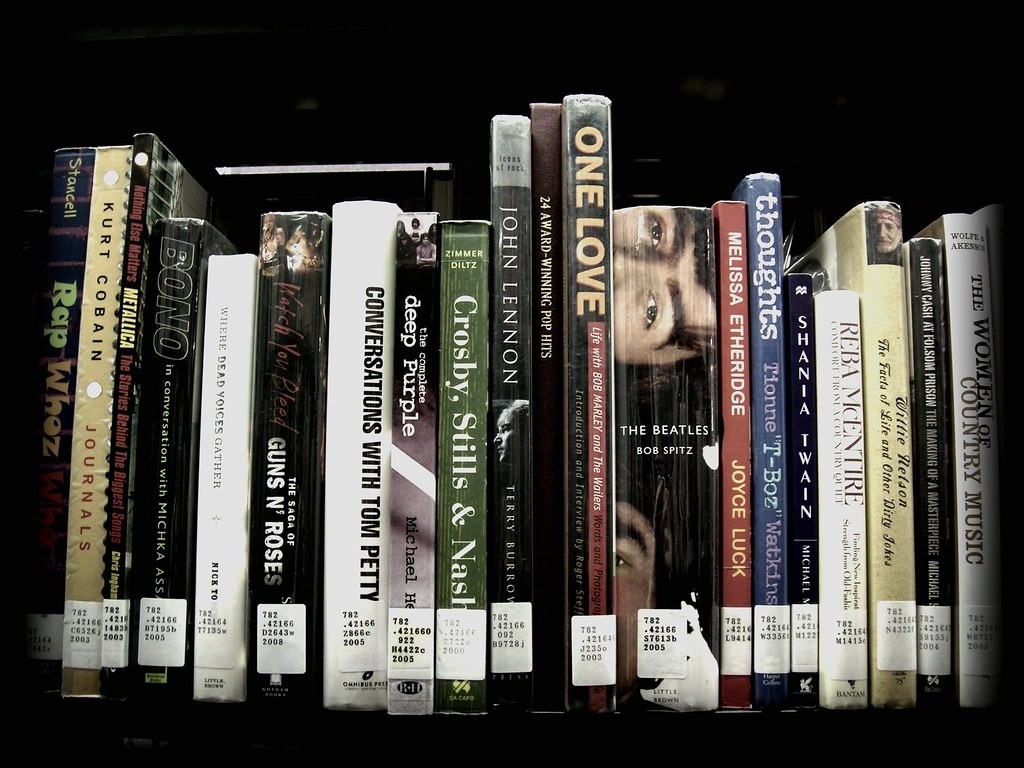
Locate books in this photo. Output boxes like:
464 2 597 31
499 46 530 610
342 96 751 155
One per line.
23 93 1004 709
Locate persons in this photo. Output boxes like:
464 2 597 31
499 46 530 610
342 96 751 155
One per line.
611 205 719 367
494 398 532 463
864 203 904 266
614 462 680 710
